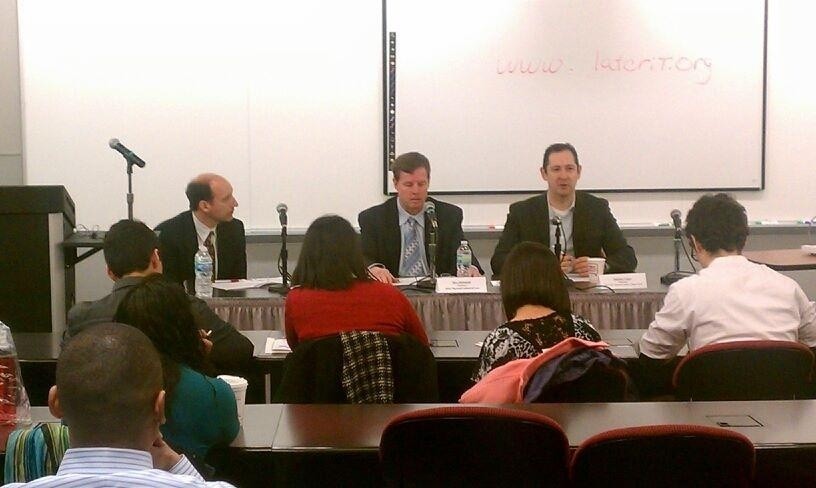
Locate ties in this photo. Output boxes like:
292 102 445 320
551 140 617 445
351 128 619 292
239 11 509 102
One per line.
205 232 216 283
403 218 426 277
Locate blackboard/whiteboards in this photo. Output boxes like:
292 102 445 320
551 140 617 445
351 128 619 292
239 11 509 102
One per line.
382 0 769 196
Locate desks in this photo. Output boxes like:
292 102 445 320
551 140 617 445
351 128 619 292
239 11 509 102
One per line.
0 404 283 488
271 398 815 487
197 275 666 328
266 330 689 404
11 328 272 403
742 248 816 271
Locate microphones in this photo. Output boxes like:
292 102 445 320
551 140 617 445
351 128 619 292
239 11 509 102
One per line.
551 215 562 225
276 203 289 238
670 209 681 232
423 200 438 230
109 138 145 169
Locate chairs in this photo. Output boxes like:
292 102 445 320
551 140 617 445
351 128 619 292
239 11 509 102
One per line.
673 339 816 402
380 407 570 487
541 361 633 401
570 422 756 488
277 334 441 402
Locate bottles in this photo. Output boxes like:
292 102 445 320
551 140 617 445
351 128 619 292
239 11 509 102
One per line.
454 240 474 286
192 247 215 302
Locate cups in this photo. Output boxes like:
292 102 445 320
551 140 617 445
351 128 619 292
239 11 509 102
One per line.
585 257 605 292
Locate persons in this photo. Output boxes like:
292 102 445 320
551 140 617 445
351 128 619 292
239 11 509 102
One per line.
62 272 239 481
61 218 255 376
490 143 637 281
358 151 485 284
631 192 816 401
443 240 601 402
152 174 247 283
0 322 238 488
283 214 430 349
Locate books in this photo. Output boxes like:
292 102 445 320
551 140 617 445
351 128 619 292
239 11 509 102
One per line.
211 276 290 291
